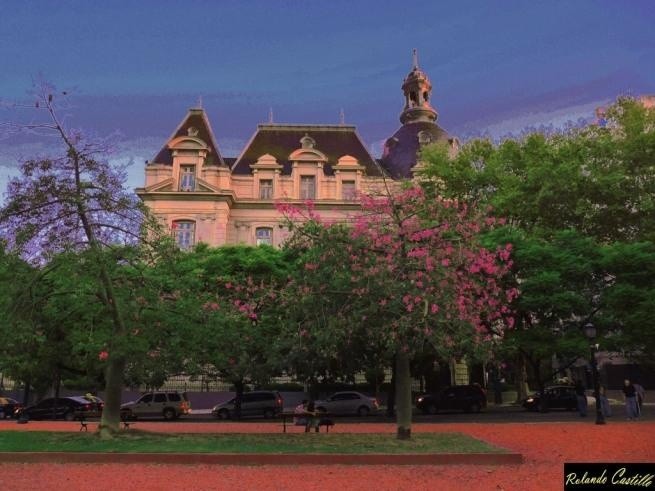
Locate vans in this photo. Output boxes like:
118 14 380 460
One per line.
212 390 284 420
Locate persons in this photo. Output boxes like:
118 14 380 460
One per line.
633 383 646 415
574 379 587 417
623 379 638 420
293 399 315 425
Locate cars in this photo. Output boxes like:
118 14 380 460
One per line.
0 397 21 421
74 394 104 418
305 390 379 418
13 396 98 423
521 385 587 414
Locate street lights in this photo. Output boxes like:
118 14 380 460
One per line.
584 323 607 425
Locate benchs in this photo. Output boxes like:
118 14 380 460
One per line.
78 410 133 431
280 411 331 432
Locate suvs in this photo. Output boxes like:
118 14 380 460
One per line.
414 383 487 415
119 391 191 421
